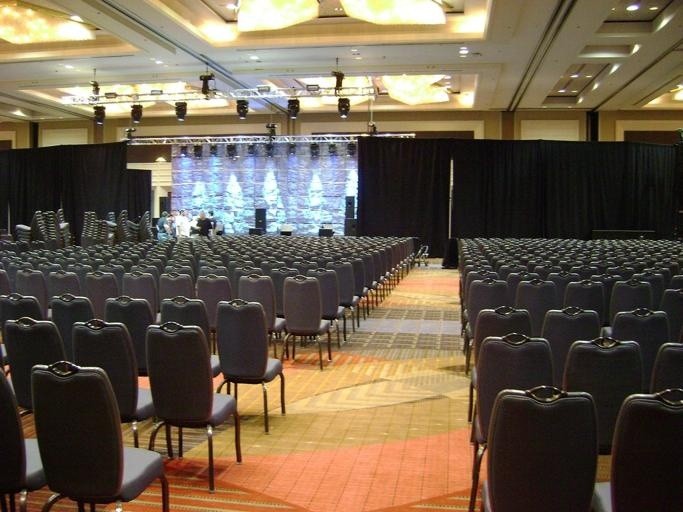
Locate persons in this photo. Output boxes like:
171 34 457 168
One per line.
156 209 225 242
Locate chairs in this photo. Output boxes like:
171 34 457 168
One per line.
1 372 85 510
70 318 174 462
30 360 170 511
144 321 244 495
483 385 599 510
468 332 557 510
648 341 682 395
562 335 647 454
456 236 682 421
215 299 287 434
2 316 67 421
2 208 429 371
608 388 682 511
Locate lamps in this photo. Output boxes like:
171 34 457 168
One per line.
88 56 378 160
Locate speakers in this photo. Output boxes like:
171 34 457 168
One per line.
318 229 334 237
249 228 266 236
345 219 357 236
255 208 266 227
346 196 354 218
